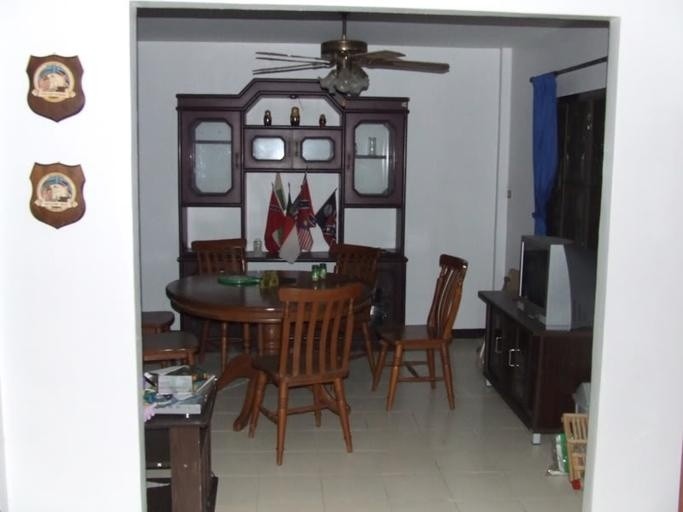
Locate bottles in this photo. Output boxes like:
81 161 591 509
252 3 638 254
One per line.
261 268 281 289
317 114 327 127
254 237 264 256
310 262 327 282
264 108 272 126
289 106 302 126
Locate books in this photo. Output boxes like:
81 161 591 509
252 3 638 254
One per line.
145 365 218 413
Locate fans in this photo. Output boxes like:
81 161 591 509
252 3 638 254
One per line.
253 15 449 74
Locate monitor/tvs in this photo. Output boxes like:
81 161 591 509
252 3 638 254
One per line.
515 234 596 331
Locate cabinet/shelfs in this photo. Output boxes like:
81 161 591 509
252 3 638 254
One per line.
477 290 592 445
144 363 217 512
175 78 409 351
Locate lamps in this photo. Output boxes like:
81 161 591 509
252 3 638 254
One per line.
320 65 368 95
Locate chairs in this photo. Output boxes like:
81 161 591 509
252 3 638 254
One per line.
372 254 468 412
142 310 199 365
328 244 381 373
190 238 250 372
248 283 364 465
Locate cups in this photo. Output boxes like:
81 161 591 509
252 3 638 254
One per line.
368 135 378 158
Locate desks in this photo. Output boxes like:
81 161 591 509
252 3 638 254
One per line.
166 270 375 431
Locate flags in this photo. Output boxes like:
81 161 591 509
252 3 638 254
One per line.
264 172 316 265
316 191 337 248
